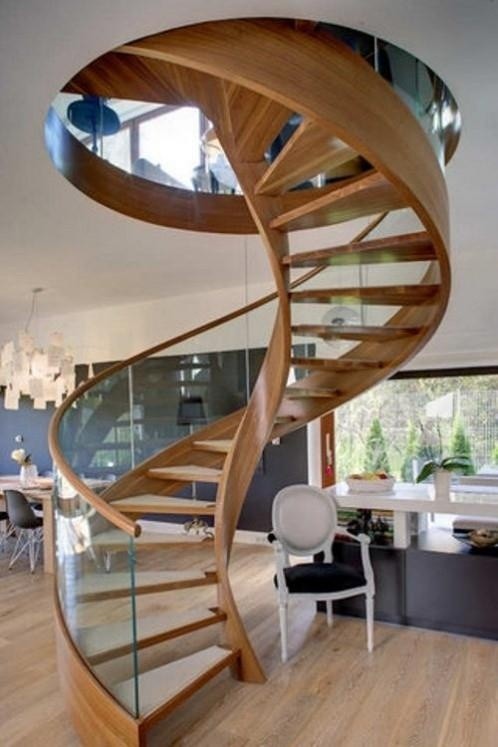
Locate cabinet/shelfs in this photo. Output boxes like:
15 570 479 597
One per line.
314 479 498 643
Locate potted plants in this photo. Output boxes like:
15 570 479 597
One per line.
415 451 475 503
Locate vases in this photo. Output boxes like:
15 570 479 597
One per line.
20 464 38 488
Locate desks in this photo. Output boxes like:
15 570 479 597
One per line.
0 474 116 538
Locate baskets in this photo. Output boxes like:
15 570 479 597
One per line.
345 473 395 493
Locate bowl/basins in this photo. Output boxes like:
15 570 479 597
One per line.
345 475 396 492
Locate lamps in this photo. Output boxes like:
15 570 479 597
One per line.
320 300 362 353
0 288 79 411
424 392 454 460
176 397 213 542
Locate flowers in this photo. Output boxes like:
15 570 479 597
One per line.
10 434 33 468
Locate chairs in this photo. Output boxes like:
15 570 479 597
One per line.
52 493 99 569
3 489 44 574
132 158 188 190
268 483 379 664
